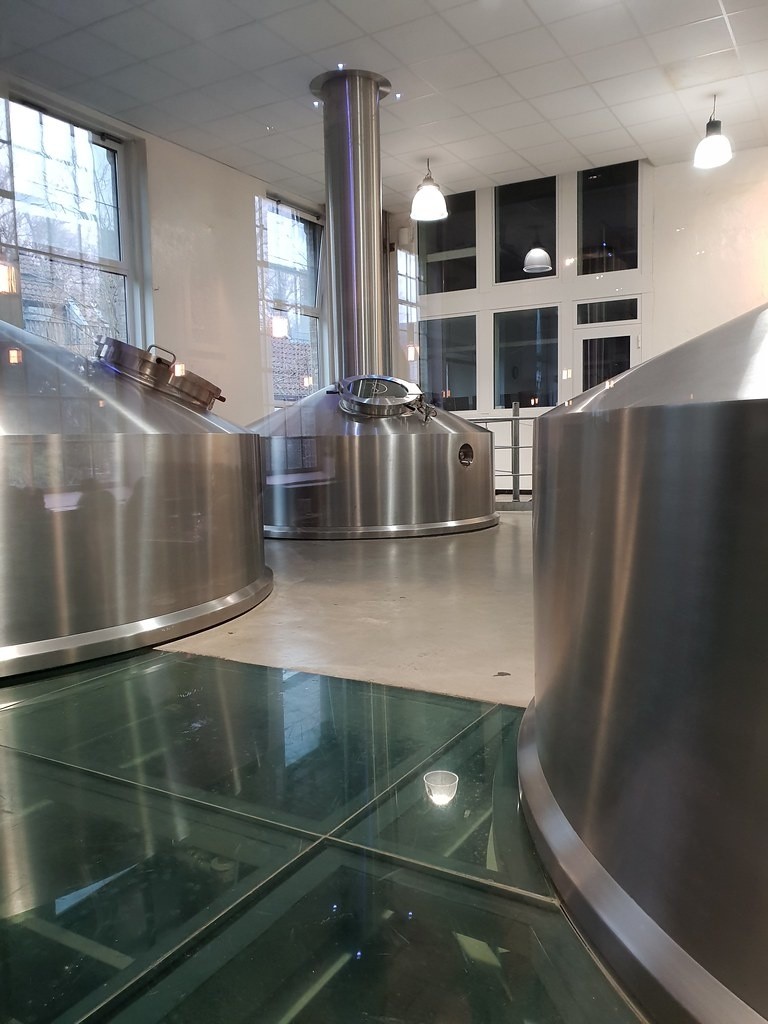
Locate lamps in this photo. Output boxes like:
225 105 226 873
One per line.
423 770 459 806
409 158 448 221
522 225 552 273
693 95 733 169
271 300 288 339
